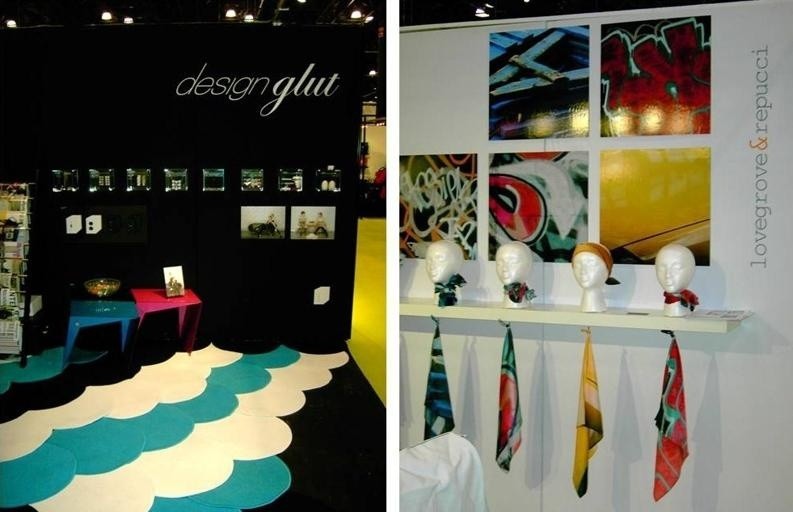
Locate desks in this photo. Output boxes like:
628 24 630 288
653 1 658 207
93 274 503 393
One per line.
54 300 138 371
129 287 203 356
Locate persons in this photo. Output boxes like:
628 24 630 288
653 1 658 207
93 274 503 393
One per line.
570 240 612 313
655 242 695 318
311 209 327 237
267 212 282 235
424 238 465 303
296 210 310 235
492 240 531 309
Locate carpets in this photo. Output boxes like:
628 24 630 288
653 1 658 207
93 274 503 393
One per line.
0 345 351 512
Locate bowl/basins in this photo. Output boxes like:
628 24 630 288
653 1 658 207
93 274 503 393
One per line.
83 279 121 297
